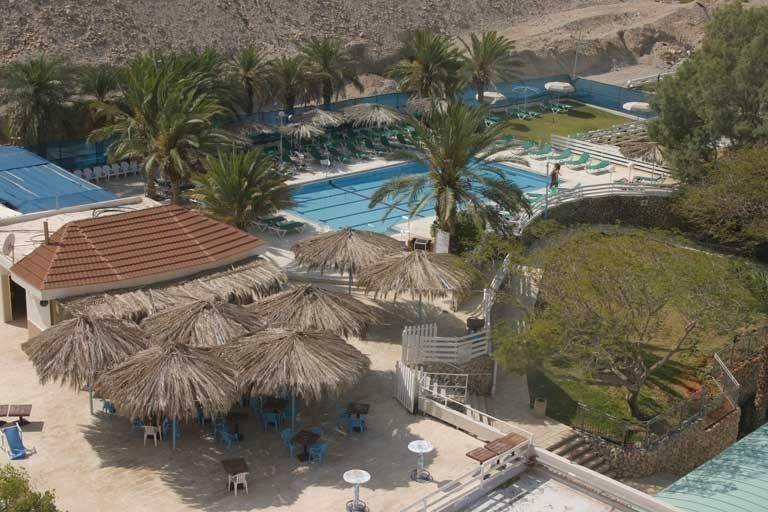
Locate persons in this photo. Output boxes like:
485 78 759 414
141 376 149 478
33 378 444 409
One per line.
548 162 561 193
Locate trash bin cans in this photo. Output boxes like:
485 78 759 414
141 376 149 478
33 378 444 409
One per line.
534 397 547 419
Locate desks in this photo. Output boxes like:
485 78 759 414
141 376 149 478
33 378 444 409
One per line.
343 467 371 511
9 404 33 427
406 440 436 484
466 431 529 474
0 404 8 427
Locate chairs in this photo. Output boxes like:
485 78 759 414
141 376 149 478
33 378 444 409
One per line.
95 393 375 492
1 423 35 461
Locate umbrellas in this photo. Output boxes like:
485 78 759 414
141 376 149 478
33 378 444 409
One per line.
545 79 577 105
476 88 506 117
623 103 652 125
